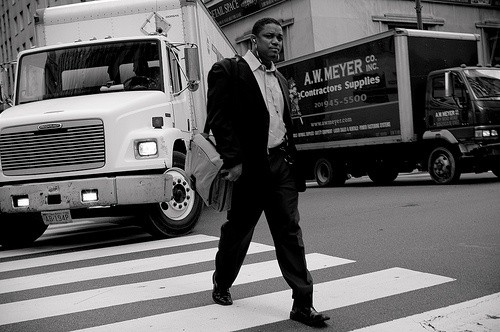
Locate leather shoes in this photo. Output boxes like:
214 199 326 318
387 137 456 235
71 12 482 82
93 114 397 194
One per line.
289 303 330 325
212 271 234 306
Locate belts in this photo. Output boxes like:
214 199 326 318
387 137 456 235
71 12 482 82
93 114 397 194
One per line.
268 141 286 154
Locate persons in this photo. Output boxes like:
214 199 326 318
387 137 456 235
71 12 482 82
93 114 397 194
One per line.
206 17 331 327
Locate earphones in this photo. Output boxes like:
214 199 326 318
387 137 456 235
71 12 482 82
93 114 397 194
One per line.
253 39 256 44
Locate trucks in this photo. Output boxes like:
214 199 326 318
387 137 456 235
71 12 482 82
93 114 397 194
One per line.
272 27 500 188
0 0 243 249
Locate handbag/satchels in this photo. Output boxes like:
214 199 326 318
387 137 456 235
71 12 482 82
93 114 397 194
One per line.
187 133 233 212
